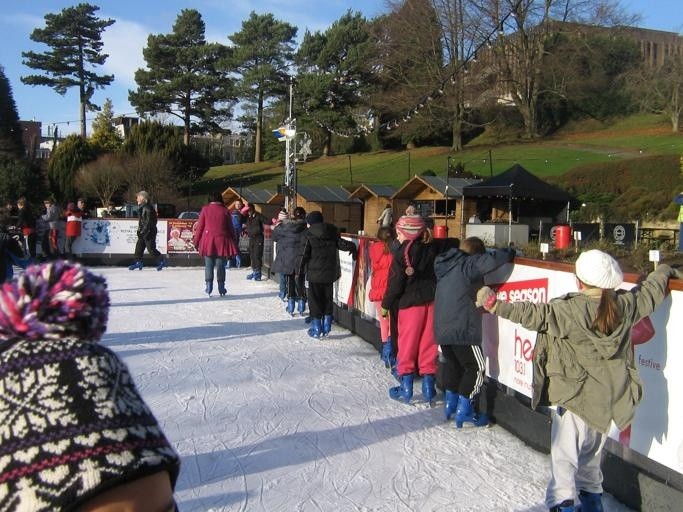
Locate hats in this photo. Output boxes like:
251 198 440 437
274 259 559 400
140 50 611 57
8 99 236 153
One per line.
278 208 288 220
397 206 426 278
241 203 255 215
376 227 397 240
67 203 76 211
575 249 624 288
44 198 54 204
306 211 323 226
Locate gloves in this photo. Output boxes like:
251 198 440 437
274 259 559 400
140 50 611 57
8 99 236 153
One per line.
381 308 389 319
475 286 499 313
140 229 145 235
295 274 305 286
348 243 357 261
674 268 682 278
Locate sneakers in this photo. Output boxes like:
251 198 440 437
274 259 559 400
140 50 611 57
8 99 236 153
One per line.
225 259 231 268
552 503 575 511
204 281 213 293
236 255 241 267
253 271 260 280
128 261 143 270
218 280 227 294
156 260 164 271
246 273 253 279
279 290 287 299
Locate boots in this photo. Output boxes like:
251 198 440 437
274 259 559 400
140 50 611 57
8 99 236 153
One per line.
285 298 328 337
389 376 495 426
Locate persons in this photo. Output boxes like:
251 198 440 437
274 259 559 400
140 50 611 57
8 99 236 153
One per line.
129 190 167 271
15 196 37 260
193 190 239 296
475 247 682 511
240 202 280 282
225 197 249 268
434 236 516 428
295 210 356 337
366 225 398 363
76 197 91 220
41 197 60 254
376 203 393 228
63 199 84 260
271 207 308 314
101 201 118 218
0 206 32 282
381 204 459 405
272 208 290 299
5 201 13 212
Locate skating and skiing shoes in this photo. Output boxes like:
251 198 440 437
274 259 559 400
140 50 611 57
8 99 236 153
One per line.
575 492 605 510
